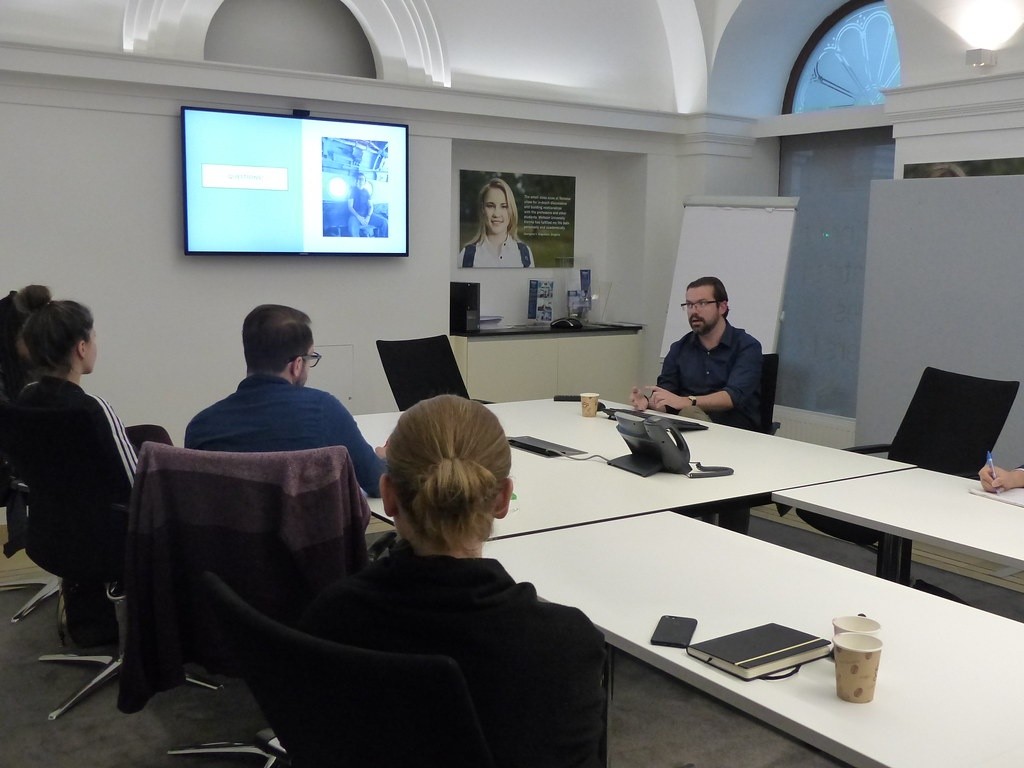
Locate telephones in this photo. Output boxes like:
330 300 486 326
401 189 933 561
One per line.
606 411 694 478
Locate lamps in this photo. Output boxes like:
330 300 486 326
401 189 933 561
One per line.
964 49 997 66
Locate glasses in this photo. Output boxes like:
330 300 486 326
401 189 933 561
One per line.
680 300 718 311
287 351 322 367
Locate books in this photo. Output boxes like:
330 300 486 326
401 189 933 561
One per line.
687 622 831 682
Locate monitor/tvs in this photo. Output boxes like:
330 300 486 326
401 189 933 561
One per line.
180 105 409 257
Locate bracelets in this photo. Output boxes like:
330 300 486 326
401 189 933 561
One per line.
643 394 649 402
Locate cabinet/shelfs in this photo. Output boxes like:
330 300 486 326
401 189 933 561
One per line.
449 324 642 403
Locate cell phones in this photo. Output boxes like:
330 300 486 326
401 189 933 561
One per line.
650 615 697 647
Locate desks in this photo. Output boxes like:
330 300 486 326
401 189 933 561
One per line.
479 509 1024 768
349 401 916 540
771 467 1024 586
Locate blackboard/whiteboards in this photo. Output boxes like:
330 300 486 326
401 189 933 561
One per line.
660 194 799 365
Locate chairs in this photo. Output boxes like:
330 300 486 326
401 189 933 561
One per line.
0 333 1019 768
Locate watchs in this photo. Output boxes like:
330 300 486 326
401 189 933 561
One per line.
688 395 697 406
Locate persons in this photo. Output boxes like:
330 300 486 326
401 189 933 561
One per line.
185 304 389 498
631 277 763 536
291 393 609 768
8 285 139 508
347 173 388 237
978 463 1024 494
458 178 535 267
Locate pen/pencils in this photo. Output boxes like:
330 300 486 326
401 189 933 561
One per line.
986 451 1000 495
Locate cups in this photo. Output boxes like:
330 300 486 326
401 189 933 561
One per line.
834 616 881 636
580 393 599 417
832 632 884 703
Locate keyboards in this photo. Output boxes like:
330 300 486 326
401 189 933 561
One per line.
603 407 709 431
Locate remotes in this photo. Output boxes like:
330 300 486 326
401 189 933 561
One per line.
554 395 581 402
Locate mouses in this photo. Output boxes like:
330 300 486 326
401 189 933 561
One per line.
597 401 606 411
550 317 583 329
